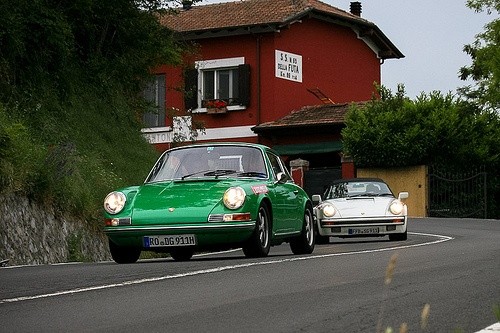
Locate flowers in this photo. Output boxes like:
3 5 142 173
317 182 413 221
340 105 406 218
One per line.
204 100 226 110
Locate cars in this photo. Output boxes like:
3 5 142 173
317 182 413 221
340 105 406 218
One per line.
312 177 408 244
102 143 315 263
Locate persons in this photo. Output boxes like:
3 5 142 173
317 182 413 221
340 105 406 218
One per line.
241 151 266 177
365 184 376 192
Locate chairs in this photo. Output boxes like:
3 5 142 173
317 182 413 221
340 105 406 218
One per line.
366 184 379 193
185 155 210 176
